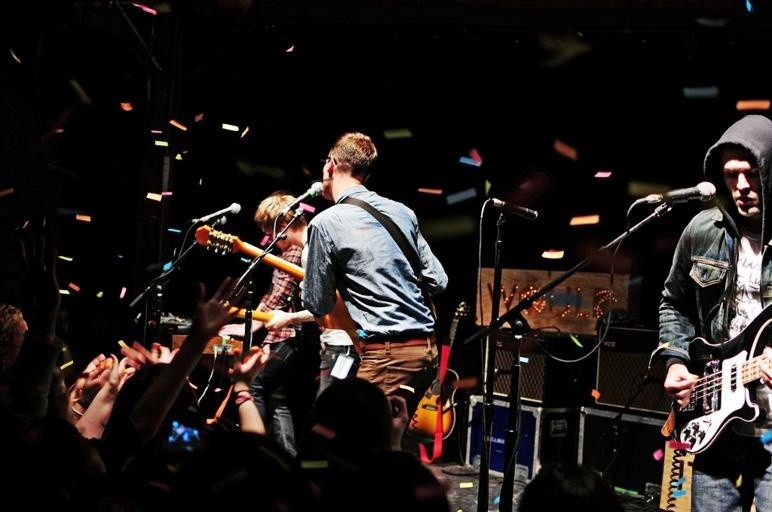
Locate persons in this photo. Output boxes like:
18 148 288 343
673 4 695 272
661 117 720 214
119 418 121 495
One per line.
304 131 450 457
3 189 637 512
657 114 772 512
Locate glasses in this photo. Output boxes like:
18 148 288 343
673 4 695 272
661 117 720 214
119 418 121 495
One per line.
319 155 339 167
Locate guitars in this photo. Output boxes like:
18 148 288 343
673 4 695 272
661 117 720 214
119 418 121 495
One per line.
194 225 362 357
408 302 470 443
670 303 772 455
226 295 321 349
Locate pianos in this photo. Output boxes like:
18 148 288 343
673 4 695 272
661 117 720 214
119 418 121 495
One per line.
172 333 253 356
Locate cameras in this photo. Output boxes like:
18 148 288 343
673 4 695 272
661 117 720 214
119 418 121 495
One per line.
166 414 212 451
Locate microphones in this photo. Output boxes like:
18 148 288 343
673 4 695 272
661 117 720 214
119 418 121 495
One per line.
193 215 227 226
283 182 323 216
491 197 539 222
636 181 716 206
201 202 241 222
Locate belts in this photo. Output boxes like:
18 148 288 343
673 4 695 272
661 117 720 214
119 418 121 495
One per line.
363 334 436 352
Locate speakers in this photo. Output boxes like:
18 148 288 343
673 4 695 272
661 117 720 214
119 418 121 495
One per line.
594 325 673 417
480 330 548 405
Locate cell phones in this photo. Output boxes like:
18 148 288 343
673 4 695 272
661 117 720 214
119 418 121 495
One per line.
243 309 252 355
